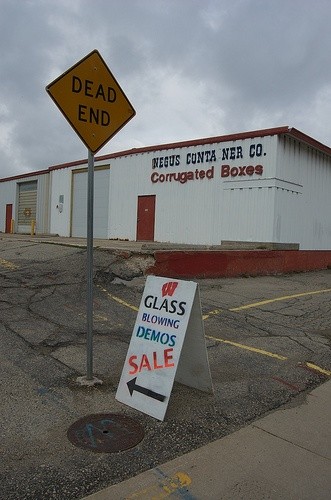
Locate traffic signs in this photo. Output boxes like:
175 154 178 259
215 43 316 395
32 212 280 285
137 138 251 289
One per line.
45 49 137 156
114 275 198 422
